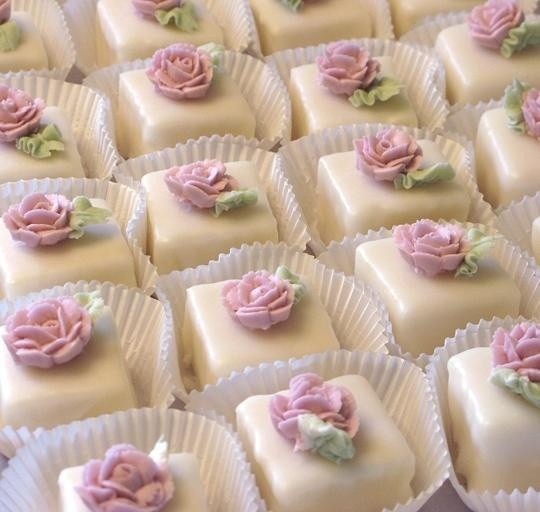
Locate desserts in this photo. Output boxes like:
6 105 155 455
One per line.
0 1 540 511
